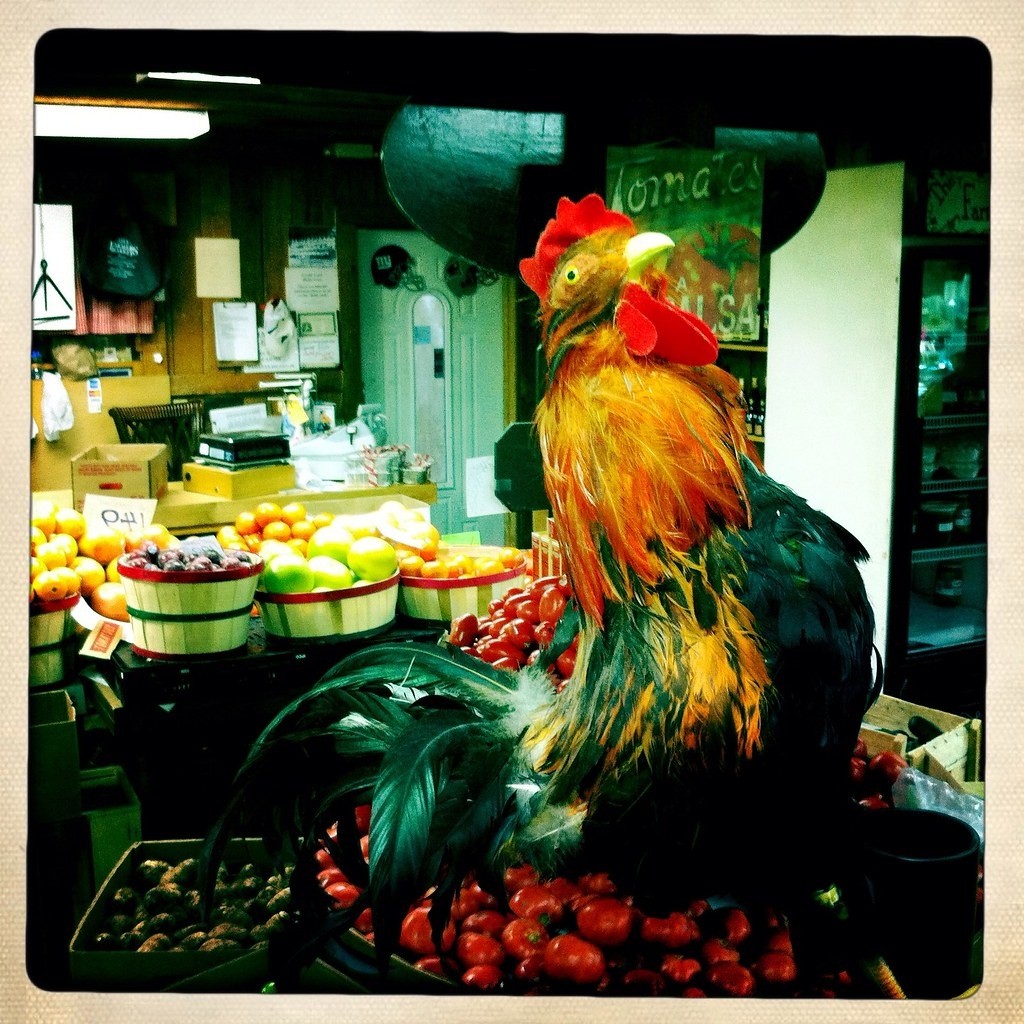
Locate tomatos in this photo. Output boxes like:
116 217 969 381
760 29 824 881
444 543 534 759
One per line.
315 575 913 999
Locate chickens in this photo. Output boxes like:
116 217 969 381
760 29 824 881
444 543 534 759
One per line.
198 192 884 984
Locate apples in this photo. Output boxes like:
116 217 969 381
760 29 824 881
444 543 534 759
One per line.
256 526 399 593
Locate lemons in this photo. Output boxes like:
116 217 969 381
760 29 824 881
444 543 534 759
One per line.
27 501 168 619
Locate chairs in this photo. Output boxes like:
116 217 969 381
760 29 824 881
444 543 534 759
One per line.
108 402 203 482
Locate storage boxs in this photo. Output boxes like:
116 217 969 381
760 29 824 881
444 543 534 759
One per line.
118 549 263 658
72 444 169 506
396 560 530 622
70 837 297 995
858 695 980 785
26 594 83 688
254 566 402 643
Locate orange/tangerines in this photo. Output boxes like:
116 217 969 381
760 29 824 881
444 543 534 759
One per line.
400 547 524 579
217 502 440 561
